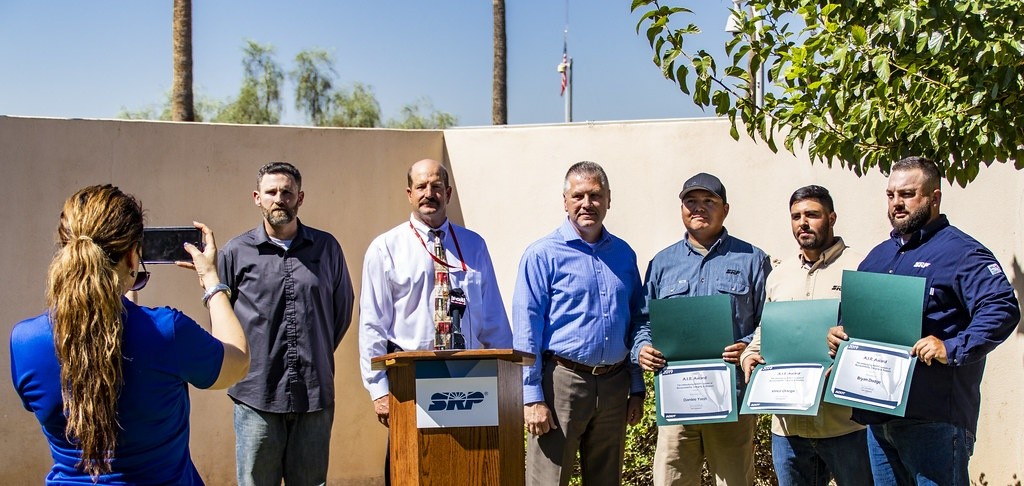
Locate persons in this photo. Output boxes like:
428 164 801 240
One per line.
358 159 513 486
630 173 773 486
740 185 865 486
218 162 355 486
828 156 1021 486
513 161 646 486
10 184 251 486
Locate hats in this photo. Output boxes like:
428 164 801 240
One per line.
679 173 726 201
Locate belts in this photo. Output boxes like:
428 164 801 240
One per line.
548 353 630 375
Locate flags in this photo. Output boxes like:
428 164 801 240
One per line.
560 42 567 96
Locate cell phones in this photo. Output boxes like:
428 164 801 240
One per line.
138 227 203 264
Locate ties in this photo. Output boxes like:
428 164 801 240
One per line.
429 229 453 349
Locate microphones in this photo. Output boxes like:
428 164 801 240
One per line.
448 290 459 298
446 288 468 350
458 288 466 297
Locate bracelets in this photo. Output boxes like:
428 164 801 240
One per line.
202 283 232 307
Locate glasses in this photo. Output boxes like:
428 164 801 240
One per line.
130 248 150 291
